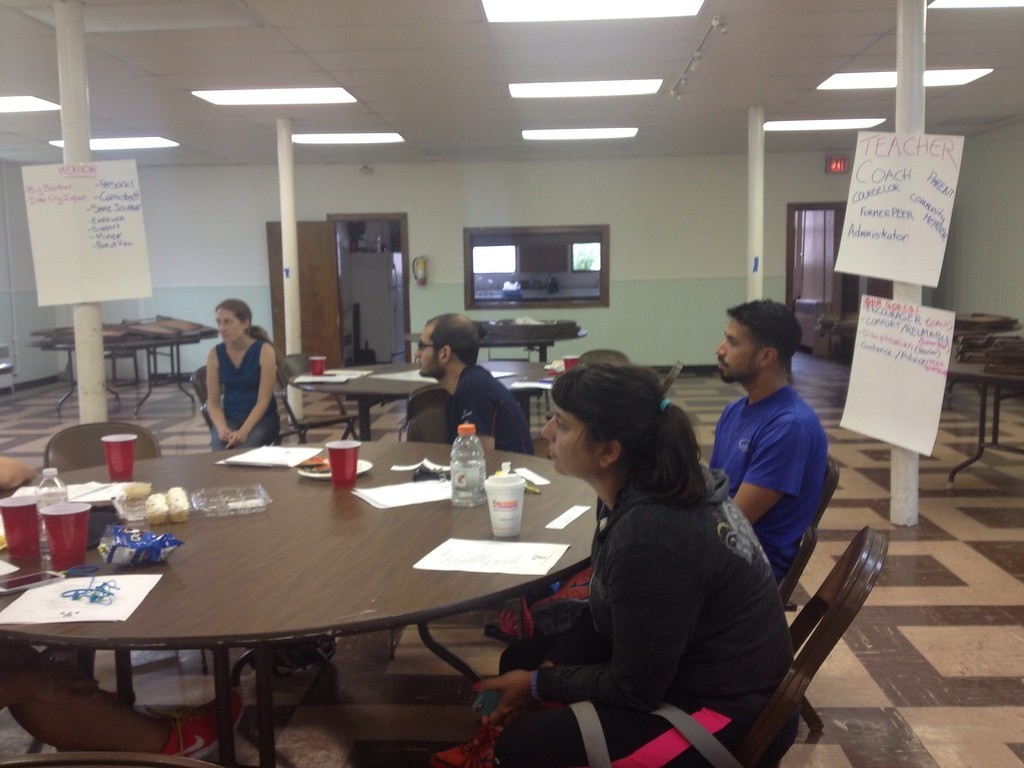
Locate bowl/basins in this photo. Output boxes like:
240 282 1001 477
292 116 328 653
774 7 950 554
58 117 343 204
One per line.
111 481 275 526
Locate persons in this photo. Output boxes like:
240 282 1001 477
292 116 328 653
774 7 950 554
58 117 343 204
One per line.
205 299 281 450
580 350 629 366
432 300 829 768
474 364 800 768
0 457 245 759
415 313 536 456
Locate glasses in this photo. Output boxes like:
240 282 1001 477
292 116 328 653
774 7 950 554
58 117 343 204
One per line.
416 340 459 357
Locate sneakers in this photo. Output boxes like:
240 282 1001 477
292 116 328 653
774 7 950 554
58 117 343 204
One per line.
96 686 135 706
162 686 245 759
430 713 504 768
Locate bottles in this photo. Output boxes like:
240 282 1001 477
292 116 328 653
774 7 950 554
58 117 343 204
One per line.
35 468 68 560
449 424 487 507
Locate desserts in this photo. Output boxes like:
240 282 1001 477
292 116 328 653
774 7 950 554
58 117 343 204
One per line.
146 486 191 526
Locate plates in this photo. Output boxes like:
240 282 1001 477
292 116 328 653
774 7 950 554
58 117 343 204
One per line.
297 457 373 480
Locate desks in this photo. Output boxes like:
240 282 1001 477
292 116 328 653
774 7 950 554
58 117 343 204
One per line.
401 325 589 364
286 360 559 442
0 440 598 768
814 313 1024 483
40 328 219 417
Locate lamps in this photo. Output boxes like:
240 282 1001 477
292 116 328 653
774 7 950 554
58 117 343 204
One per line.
670 18 728 100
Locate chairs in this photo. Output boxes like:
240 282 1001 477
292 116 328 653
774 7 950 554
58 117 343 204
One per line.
737 526 889 767
775 459 841 732
272 354 358 443
396 384 452 443
189 364 214 427
42 423 208 675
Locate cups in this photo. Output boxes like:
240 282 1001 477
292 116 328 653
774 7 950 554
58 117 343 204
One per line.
0 495 41 559
309 357 326 376
101 434 138 483
325 440 362 489
483 473 526 536
38 502 92 571
563 355 580 370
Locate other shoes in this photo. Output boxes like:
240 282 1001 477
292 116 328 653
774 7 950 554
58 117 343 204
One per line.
529 565 594 637
247 634 337 671
